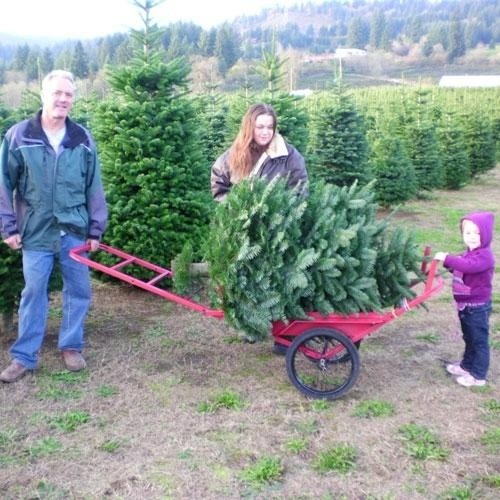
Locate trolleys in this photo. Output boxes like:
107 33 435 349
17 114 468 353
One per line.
64 234 450 403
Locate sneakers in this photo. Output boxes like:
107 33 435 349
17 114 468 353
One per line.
456 373 486 387
61 349 85 372
446 363 465 375
1 360 29 383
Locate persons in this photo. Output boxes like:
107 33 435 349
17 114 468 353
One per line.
211 103 308 204
0 70 108 383
434 211 495 386
